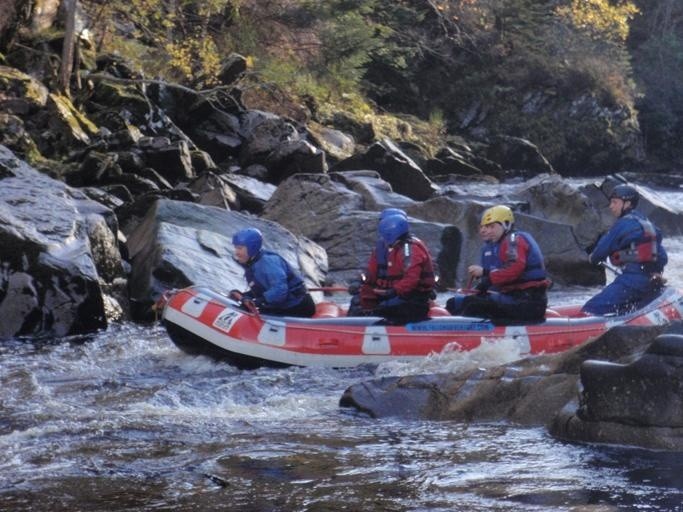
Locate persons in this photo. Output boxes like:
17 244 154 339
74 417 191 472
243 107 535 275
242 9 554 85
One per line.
578 184 667 319
225 225 316 318
344 206 408 317
457 205 551 327
370 215 438 323
444 208 499 320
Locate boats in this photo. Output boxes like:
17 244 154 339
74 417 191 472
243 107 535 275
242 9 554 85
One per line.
162 284 683 370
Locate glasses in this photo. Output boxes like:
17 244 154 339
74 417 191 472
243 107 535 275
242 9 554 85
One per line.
608 183 640 209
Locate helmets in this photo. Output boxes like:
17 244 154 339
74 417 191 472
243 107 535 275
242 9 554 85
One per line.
231 228 263 258
477 207 492 223
378 208 408 222
377 213 410 247
484 204 515 226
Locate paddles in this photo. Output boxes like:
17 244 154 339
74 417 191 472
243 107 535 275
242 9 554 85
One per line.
231 290 263 324
